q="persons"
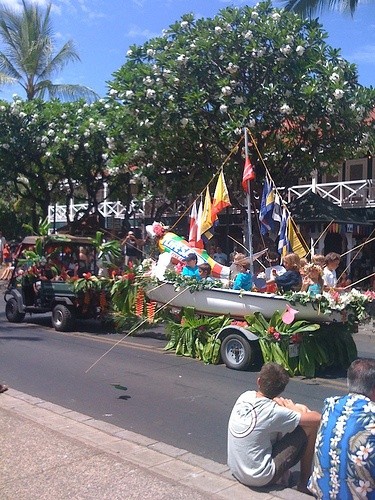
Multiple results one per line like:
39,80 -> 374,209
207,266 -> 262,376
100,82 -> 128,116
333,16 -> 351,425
182,254 -> 214,283
80,247 -> 87,261
230,253 -> 251,291
305,358 -> 375,500
213,247 -> 227,266
227,362 -> 322,496
267,253 -> 302,294
0,231 -> 16,268
123,231 -> 140,265
299,253 -> 351,297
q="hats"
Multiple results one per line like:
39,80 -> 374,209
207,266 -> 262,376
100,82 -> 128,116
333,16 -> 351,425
265,252 -> 279,262
235,260 -> 248,270
182,253 -> 197,261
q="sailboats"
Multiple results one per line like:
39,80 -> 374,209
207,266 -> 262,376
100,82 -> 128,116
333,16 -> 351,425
135,127 -> 355,324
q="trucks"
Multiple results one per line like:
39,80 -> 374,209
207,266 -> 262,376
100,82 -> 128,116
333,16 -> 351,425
4,233 -> 116,333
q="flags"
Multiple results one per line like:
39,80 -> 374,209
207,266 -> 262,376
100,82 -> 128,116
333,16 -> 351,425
259,174 -> 289,266
188,171 -> 232,248
242,157 -> 255,193
